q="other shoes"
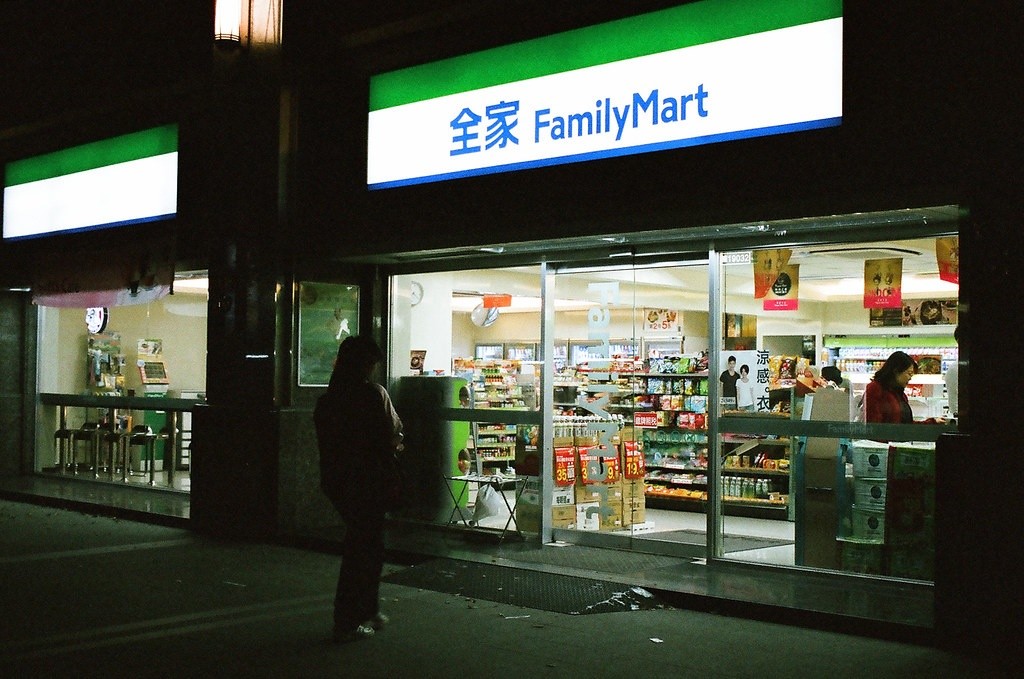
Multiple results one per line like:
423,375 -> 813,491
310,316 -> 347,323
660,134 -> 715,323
362,612 -> 388,628
332,625 -> 375,642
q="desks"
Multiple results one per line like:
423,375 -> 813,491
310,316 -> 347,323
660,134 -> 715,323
443,474 -> 529,547
39,393 -> 203,485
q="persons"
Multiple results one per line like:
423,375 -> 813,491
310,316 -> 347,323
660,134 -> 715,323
735,364 -> 754,411
314,335 -> 404,640
866,351 -> 946,423
720,356 -> 740,409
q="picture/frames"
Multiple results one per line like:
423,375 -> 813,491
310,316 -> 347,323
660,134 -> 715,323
141,361 -> 169,383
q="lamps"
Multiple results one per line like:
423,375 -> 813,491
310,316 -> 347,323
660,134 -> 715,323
334,309 -> 346,326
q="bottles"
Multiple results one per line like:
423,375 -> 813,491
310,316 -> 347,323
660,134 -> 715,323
482,368 -> 504,382
720,476 -> 773,500
553,413 -> 626,438
835,358 -> 886,372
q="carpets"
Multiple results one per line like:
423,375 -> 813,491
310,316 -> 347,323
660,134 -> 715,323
633,528 -> 795,555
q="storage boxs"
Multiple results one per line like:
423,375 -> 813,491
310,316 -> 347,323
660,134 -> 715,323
515,427 -> 645,533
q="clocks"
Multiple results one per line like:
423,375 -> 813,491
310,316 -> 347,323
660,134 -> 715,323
411,281 -> 423,306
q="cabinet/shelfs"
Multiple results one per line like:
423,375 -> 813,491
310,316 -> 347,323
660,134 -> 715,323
454,358 -> 643,488
552,374 -> 793,520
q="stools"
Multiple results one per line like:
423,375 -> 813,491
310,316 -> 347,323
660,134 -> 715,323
73,422 -> 120,478
129,426 -> 178,486
105,424 -> 152,482
55,421 -> 99,476
